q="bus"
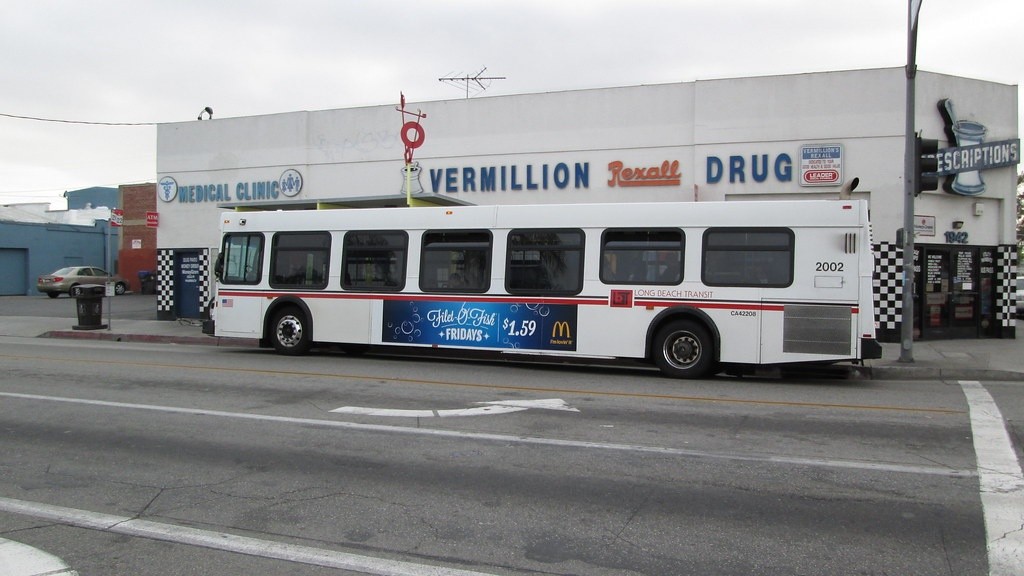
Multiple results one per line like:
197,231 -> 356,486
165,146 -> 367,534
214,199 -> 884,379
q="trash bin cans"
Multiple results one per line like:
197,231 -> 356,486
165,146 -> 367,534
139,271 -> 157,294
71,284 -> 108,329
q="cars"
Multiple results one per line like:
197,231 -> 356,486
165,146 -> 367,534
37,266 -> 130,298
